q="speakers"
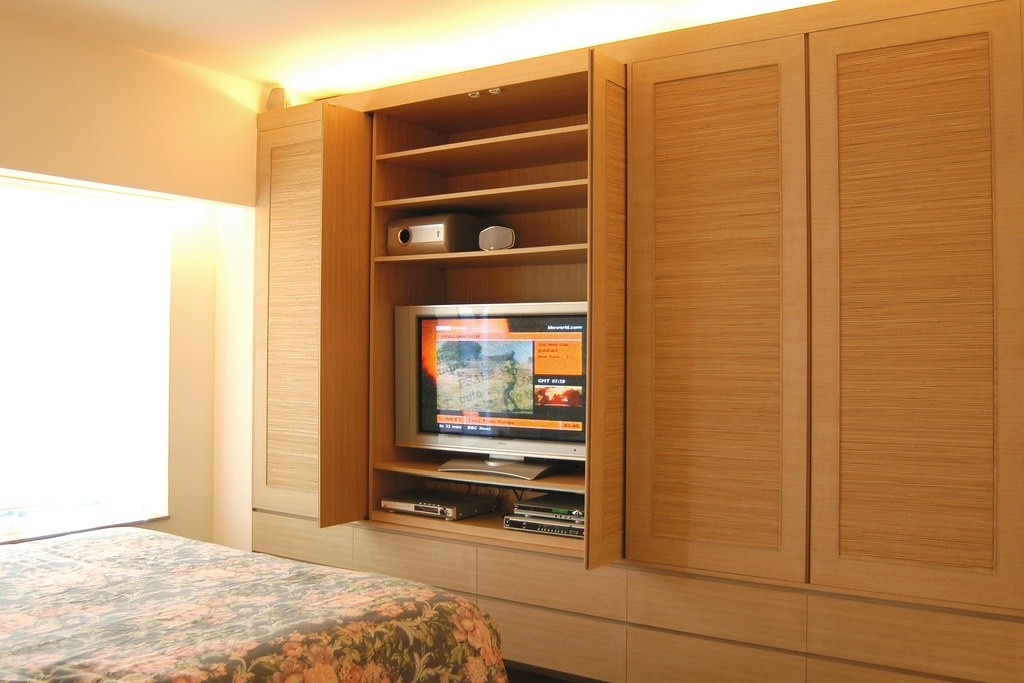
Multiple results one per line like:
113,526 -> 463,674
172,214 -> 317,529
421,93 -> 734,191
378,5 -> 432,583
478,226 -> 515,251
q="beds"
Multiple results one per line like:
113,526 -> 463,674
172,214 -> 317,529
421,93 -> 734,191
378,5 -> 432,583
0,527 -> 506,683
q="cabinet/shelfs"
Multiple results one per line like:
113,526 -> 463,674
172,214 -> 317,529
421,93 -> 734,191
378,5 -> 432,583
251,1 -> 1024,683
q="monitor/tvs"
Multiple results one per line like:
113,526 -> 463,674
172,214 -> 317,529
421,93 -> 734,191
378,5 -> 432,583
393,302 -> 588,480
